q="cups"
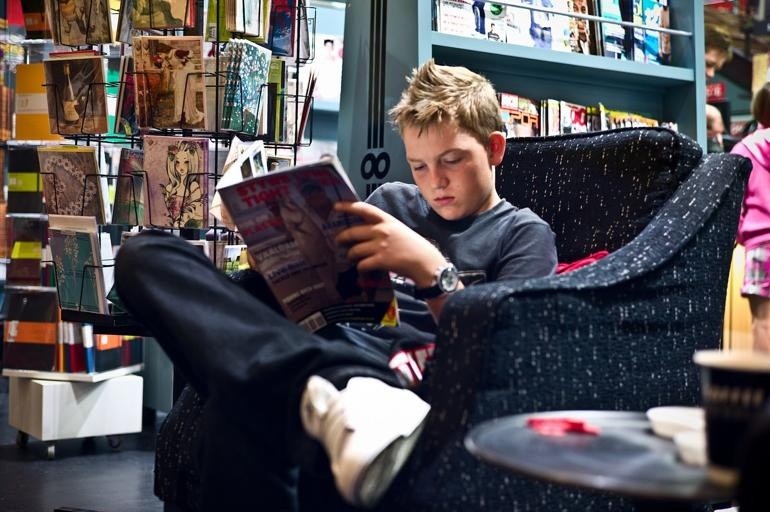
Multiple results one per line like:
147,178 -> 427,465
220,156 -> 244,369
691,348 -> 770,488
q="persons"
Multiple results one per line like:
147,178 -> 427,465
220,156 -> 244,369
702,22 -> 735,148
729,80 -> 770,355
295,174 -> 381,305
114,58 -> 556,510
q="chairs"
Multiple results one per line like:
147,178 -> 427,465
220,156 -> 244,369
149,124 -> 755,509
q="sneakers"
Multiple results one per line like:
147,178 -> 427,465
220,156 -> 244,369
299,372 -> 431,507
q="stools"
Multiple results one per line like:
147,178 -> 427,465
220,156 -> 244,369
7,372 -> 143,461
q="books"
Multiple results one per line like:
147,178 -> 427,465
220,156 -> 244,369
218,140 -> 400,334
433,1 -> 690,146
0,1 -> 318,375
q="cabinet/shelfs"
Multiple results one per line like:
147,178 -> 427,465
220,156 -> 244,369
337,0 -> 710,201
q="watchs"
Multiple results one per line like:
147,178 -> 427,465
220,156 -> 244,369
412,262 -> 459,302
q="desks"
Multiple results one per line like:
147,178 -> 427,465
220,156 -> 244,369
462,407 -> 768,510
